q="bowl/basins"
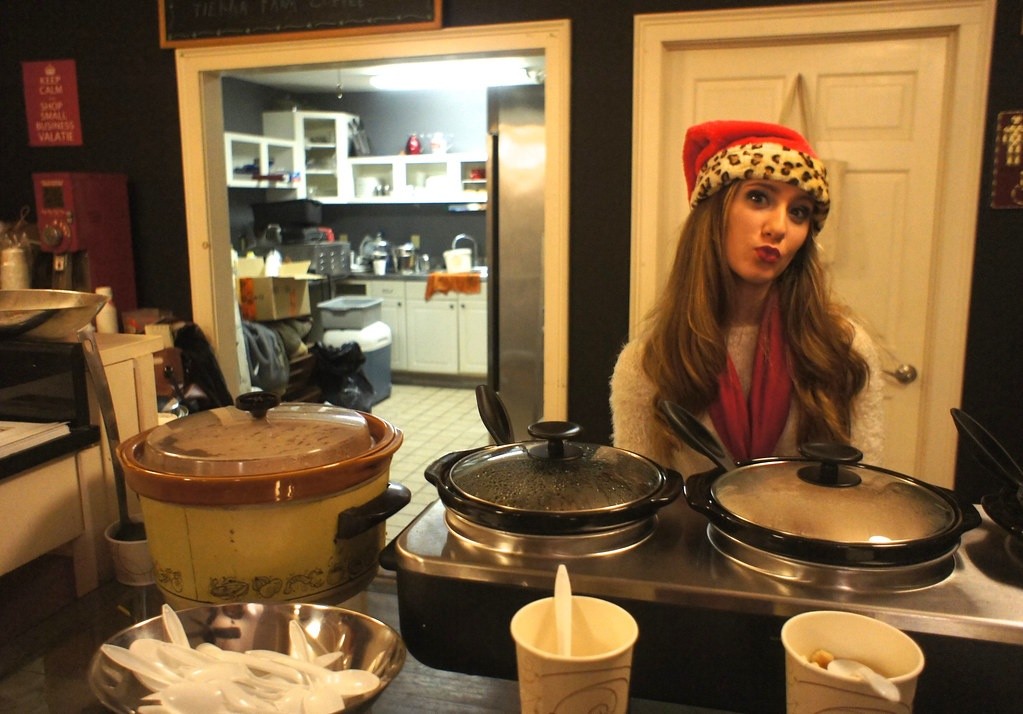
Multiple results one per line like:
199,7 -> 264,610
88,601 -> 407,713
0,289 -> 109,341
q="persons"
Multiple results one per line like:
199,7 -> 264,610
608,122 -> 883,483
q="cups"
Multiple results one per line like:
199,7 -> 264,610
355,177 -> 381,198
781,609 -> 926,714
442,248 -> 472,274
471,169 -> 485,178
510,596 -> 639,714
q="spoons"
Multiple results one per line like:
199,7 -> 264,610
99,603 -> 380,713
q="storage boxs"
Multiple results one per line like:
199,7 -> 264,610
234,255 -> 324,320
122,307 -> 184,395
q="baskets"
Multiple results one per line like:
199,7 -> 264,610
298,241 -> 351,275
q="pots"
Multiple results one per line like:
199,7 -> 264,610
424,383 -> 685,537
657,401 -> 983,568
116,391 -> 413,607
950,407 -> 1023,540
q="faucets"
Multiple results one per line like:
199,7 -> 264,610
450,232 -> 479,267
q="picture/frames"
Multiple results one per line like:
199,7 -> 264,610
158,0 -> 443,51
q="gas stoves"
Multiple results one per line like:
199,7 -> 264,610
377,495 -> 1023,708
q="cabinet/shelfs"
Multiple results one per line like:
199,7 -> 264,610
329,278 -> 405,373
406,280 -> 489,378
224,111 -> 486,207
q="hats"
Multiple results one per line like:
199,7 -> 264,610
682,119 -> 831,237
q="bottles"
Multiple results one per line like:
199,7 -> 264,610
370,231 -> 430,277
407,134 -> 421,154
94,287 -> 120,334
